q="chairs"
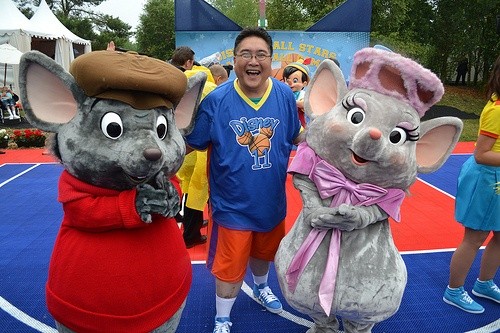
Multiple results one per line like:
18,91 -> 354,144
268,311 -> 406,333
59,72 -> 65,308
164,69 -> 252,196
0,107 -> 22,123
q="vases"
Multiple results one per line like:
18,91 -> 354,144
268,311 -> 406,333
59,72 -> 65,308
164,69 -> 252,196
36,139 -> 44,147
16,140 -> 23,146
24,141 -> 31,147
31,138 -> 36,145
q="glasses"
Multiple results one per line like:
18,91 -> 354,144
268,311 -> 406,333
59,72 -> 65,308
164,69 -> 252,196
237,52 -> 271,60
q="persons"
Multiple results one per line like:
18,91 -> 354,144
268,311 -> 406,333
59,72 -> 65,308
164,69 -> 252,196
281,58 -> 312,130
170,27 -> 303,333
444,62 -> 500,314
0,86 -> 20,120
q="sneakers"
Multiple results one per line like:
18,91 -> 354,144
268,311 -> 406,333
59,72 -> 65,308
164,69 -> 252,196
472,278 -> 500,303
443,284 -> 484,314
213,315 -> 232,333
253,282 -> 282,314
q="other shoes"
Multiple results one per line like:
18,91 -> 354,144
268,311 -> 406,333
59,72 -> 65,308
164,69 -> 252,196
9,114 -> 21,120
186,235 -> 208,249
202,219 -> 209,227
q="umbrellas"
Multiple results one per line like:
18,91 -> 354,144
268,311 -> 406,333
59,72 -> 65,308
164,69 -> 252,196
0,42 -> 26,87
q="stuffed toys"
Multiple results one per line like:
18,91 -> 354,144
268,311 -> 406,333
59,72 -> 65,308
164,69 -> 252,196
19,51 -> 206,332
276,49 -> 464,332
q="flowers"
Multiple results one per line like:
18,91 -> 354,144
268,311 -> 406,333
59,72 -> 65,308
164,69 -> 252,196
12,129 -> 43,138
